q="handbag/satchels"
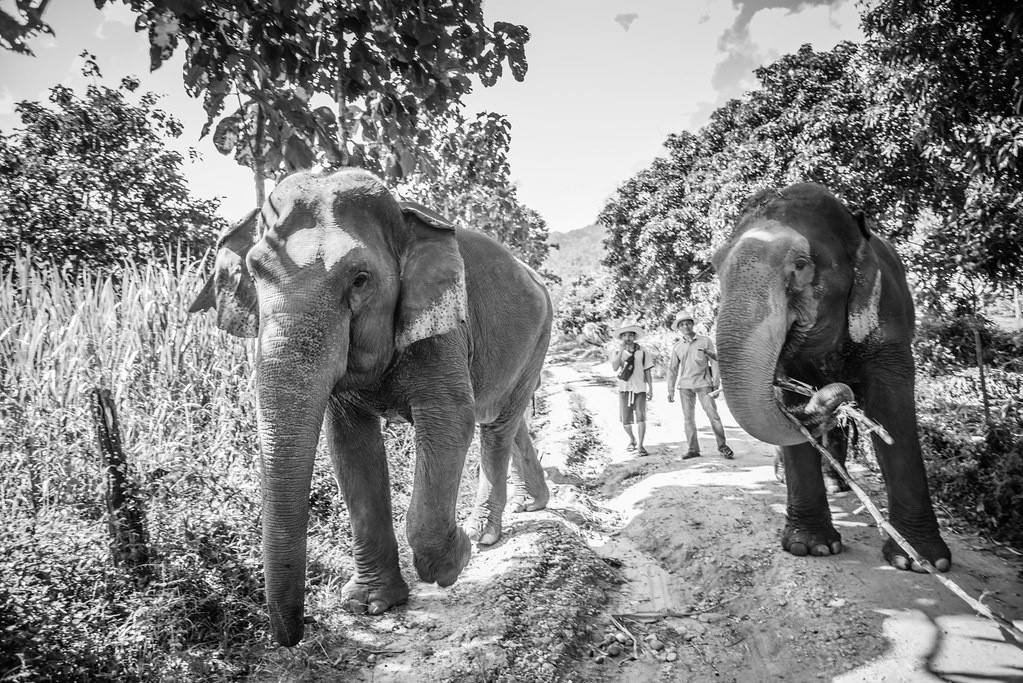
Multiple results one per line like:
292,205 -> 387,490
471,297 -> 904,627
616,356 -> 636,381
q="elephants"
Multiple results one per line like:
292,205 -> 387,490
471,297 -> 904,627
187,171 -> 554,649
711,183 -> 954,573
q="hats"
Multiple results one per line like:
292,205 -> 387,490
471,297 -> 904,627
613,320 -> 645,341
671,310 -> 699,330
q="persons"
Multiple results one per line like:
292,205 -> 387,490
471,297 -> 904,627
667,311 -> 734,459
612,318 -> 655,457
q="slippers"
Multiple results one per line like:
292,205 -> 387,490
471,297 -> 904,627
681,450 -> 700,459
637,446 -> 648,457
719,445 -> 734,458
626,441 -> 637,452
